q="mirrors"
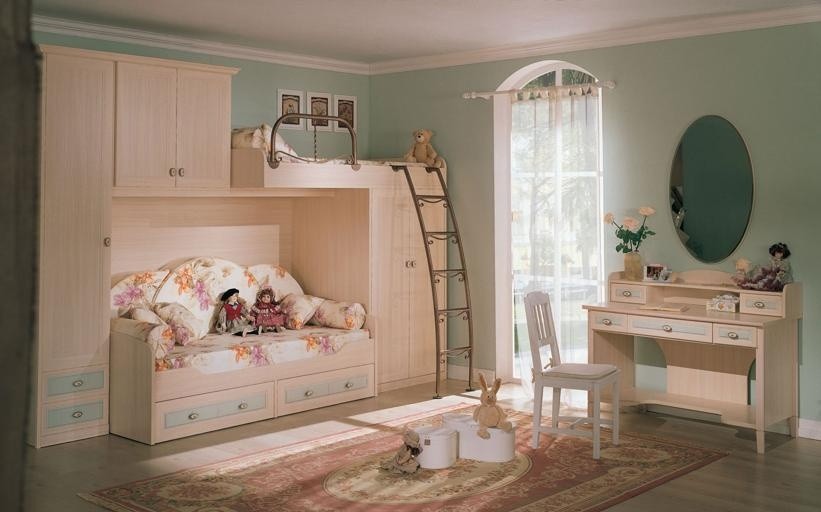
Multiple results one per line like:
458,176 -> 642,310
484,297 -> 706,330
669,114 -> 754,266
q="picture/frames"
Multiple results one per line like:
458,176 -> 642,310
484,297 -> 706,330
276,87 -> 304,130
333,94 -> 358,133
306,91 -> 331,133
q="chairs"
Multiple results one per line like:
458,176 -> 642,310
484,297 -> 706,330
523,290 -> 621,459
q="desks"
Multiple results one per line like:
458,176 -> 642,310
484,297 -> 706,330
582,269 -> 803,455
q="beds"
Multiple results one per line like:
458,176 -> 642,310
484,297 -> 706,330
231,114 -> 445,169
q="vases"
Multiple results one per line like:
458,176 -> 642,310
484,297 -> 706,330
624,253 -> 643,281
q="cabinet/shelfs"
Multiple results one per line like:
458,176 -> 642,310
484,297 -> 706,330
114,50 -> 241,190
24,44 -> 114,450
373,184 -> 445,385
109,341 -> 373,446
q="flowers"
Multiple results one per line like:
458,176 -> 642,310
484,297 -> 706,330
603,205 -> 657,254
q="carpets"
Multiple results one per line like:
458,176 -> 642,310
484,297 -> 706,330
76,400 -> 728,512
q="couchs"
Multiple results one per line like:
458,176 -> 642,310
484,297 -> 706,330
110,257 -> 370,376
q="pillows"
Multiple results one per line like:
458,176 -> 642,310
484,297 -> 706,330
128,304 -> 179,359
277,292 -> 324,332
308,298 -> 365,330
231,123 -> 298,157
154,303 -> 206,344
